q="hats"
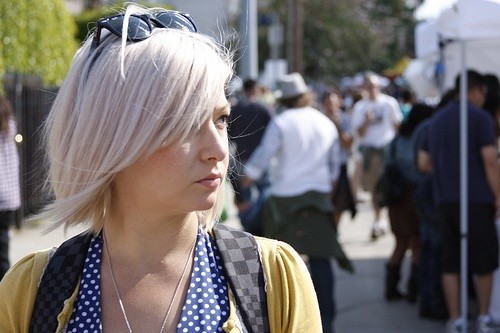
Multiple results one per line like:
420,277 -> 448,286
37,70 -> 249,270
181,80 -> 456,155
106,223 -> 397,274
357,72 -> 381,87
277,70 -> 312,101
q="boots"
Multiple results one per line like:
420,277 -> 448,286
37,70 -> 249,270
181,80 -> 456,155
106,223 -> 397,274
386,264 -> 405,300
405,270 -> 421,304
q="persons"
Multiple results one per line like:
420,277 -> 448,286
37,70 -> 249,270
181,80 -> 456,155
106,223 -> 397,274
320,92 -> 357,228
344,71 -> 404,238
0,89 -> 23,281
227,76 -> 273,229
243,73 -> 342,333
0,4 -> 323,333
373,69 -> 500,333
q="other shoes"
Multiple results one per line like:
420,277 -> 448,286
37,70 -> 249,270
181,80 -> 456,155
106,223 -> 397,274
474,314 -> 498,332
444,317 -> 463,333
371,228 -> 385,239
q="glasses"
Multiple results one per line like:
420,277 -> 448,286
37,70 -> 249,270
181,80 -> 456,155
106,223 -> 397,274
90,11 -> 199,50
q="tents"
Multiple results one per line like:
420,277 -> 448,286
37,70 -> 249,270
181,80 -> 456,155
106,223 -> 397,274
403,0 -> 500,333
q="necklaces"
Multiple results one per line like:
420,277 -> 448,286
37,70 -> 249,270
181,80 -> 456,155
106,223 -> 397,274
102,230 -> 196,333
